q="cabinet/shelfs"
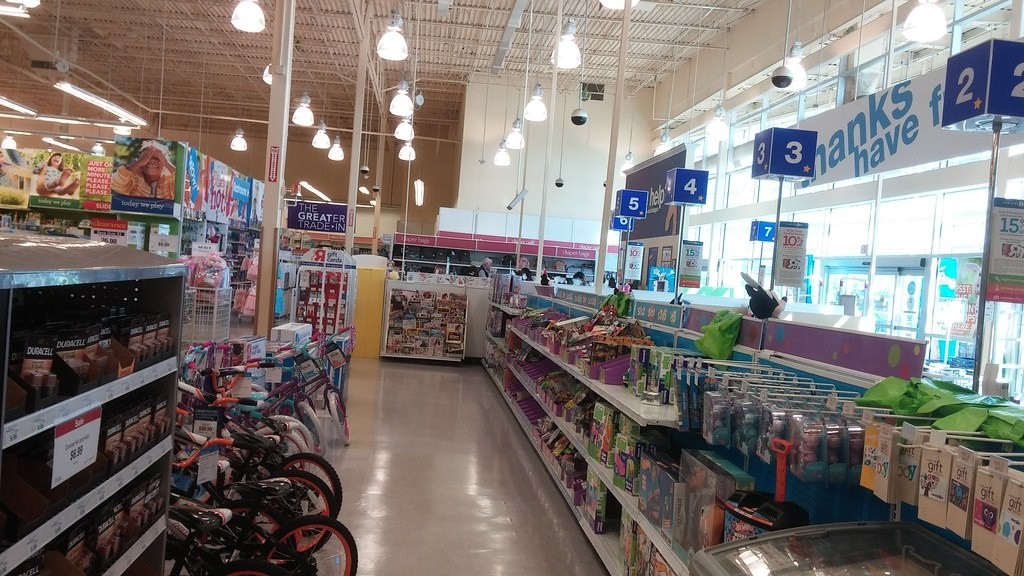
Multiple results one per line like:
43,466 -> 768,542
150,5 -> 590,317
481,273 -> 1024,576
0,136 -> 357,410
0,232 -> 188,576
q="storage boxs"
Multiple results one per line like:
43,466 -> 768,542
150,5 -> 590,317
486,273 -> 528,392
649,543 -> 678,576
613,412 -> 686,550
584,465 -> 607,534
588,401 -> 621,468
627,345 -> 714,406
230,335 -> 266,382
270,322 -> 312,346
616,505 -> 652,576
0,336 -> 173,576
672,449 -> 756,569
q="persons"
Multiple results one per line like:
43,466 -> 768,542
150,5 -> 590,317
473,257 -> 493,278
515,257 -> 533,281
35,153 -> 81,197
110,140 -> 177,200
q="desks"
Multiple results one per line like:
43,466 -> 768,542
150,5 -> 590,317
380,288 -> 469,368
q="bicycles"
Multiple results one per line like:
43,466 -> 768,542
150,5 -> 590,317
162,322 -> 360,576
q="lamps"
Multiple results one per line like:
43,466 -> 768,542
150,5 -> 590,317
494,50 -> 511,166
394,115 -> 414,140
230,47 -> 247,151
902,0 -> 947,42
376,0 -> 409,60
389,60 -> 413,116
705,11 -> 730,141
523,0 -> 547,122
0,0 -> 149,156
328,105 -> 344,161
551,0 -> 581,68
413,144 -> 424,207
620,74 -> 637,179
507,89 -> 524,149
653,6 -> 683,157
782,0 -> 808,92
231,0 -> 265,33
312,60 -> 330,149
291,16 -> 315,126
398,140 -> 416,161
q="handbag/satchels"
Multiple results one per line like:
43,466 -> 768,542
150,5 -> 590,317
599,292 -> 632,316
695,308 -> 745,371
